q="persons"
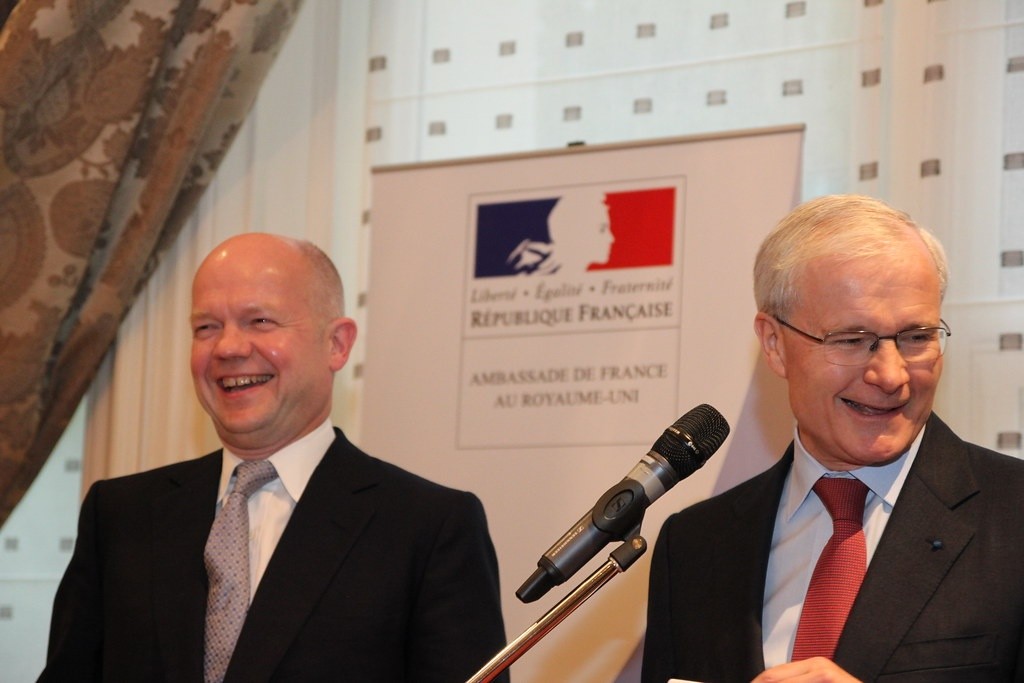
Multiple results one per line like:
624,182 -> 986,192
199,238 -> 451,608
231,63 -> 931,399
34,231 -> 511,682
640,193 -> 1024,682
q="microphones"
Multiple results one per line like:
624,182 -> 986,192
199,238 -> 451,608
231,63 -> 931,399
515,402 -> 730,603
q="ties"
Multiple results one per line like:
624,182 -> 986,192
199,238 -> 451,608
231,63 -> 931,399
792,477 -> 870,661
202,457 -> 279,683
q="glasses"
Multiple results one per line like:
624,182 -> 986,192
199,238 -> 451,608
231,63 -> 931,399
773,311 -> 951,366
